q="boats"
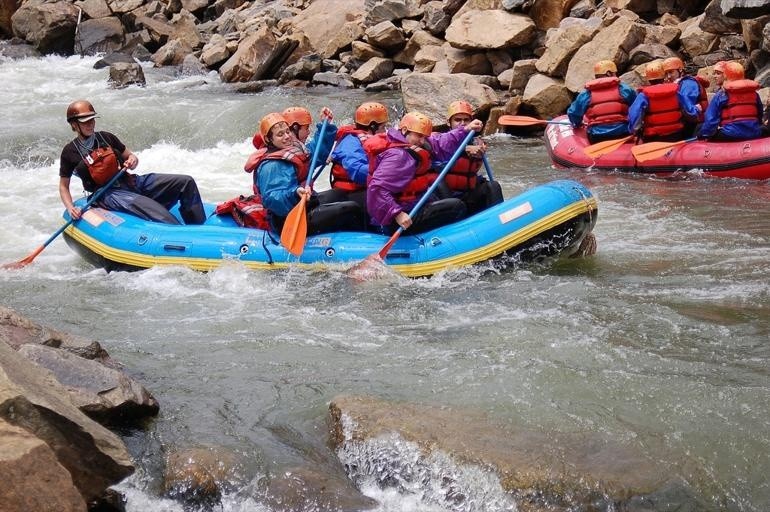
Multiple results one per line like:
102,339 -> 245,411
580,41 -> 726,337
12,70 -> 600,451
543,115 -> 770,182
60,177 -> 600,279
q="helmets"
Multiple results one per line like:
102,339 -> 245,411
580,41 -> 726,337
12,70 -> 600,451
713,60 -> 744,80
282,107 -> 311,127
663,58 -> 684,71
645,61 -> 665,80
67,100 -> 97,121
356,101 -> 388,126
260,113 -> 288,145
398,112 -> 432,137
448,101 -> 473,120
595,60 -> 618,75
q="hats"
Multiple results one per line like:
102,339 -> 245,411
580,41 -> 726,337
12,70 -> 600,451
75,115 -> 100,122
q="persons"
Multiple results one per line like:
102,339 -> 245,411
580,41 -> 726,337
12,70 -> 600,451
565,59 -> 638,142
245,102 -> 503,237
711,59 -> 726,91
699,59 -> 766,142
662,56 -> 711,123
60,100 -> 205,225
628,59 -> 702,139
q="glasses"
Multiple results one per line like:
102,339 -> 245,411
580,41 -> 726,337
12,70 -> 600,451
453,117 -> 471,122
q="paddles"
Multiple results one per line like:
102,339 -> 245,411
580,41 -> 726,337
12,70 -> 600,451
278,107 -> 334,258
632,134 -> 698,163
497,114 -> 587,126
1,155 -> 129,273
585,131 -> 639,160
346,128 -> 476,288
